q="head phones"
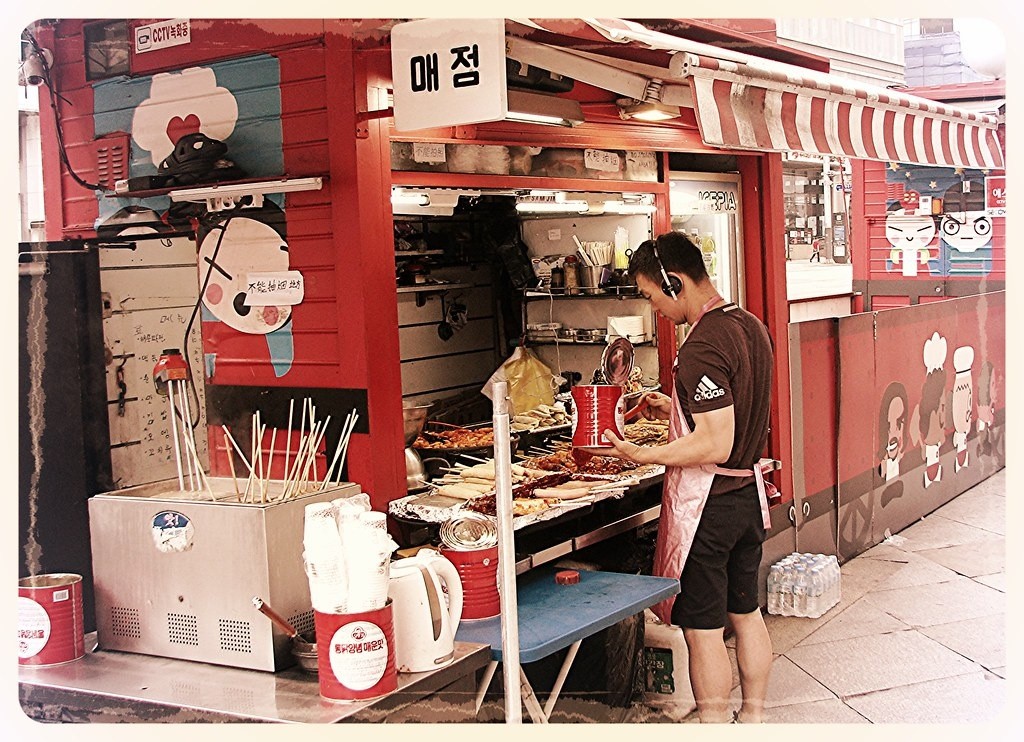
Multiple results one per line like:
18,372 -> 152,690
652,242 -> 683,301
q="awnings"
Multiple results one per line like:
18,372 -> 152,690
507,18 -> 1005,170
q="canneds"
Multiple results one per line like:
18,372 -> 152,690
18,573 -> 87,667
570,338 -> 634,448
437,511 -> 502,623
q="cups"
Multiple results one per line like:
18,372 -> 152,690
18,573 -> 85,666
314,597 -> 399,702
305,498 -> 389,612
579,262 -> 612,293
614,268 -> 638,293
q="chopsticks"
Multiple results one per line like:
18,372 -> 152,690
572,234 -> 614,266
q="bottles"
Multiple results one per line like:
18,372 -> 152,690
678,228 -> 717,277
549,253 -> 578,297
417,238 -> 428,253
767,551 -> 843,618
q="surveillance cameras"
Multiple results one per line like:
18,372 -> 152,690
25,60 -> 46,86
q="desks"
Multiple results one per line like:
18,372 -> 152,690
18,642 -> 493,724
455,566 -> 681,723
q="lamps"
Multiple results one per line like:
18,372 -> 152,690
626,103 -> 681,121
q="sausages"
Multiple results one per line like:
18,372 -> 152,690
532,480 -> 610,499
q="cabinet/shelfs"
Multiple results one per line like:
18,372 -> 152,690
519,289 -> 654,348
395,248 -> 473,294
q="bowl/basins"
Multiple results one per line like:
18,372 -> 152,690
401,400 -> 435,448
291,631 -> 318,674
528,324 -> 607,342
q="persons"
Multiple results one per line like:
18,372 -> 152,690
809,238 -> 821,263
580,230 -> 782,722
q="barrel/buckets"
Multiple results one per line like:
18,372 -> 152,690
643,617 -> 697,712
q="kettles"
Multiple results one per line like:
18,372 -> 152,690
387,555 -> 463,670
